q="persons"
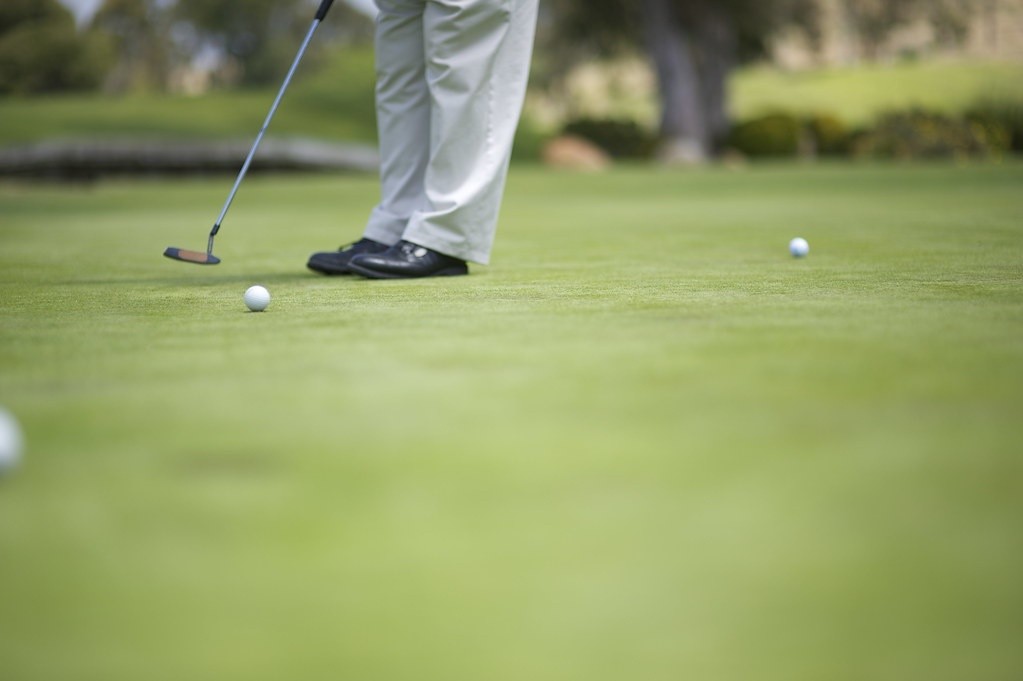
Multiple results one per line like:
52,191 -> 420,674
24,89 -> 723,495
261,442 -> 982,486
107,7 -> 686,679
637,0 -> 728,166
306,0 -> 541,279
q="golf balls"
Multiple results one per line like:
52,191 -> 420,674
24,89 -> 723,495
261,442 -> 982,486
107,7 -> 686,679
789,237 -> 810,257
244,285 -> 270,312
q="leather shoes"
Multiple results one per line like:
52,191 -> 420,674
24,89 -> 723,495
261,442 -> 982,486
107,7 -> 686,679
346,239 -> 467,279
307,237 -> 391,275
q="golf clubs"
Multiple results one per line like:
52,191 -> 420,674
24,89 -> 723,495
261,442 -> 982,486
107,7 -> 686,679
163,0 -> 333,266
